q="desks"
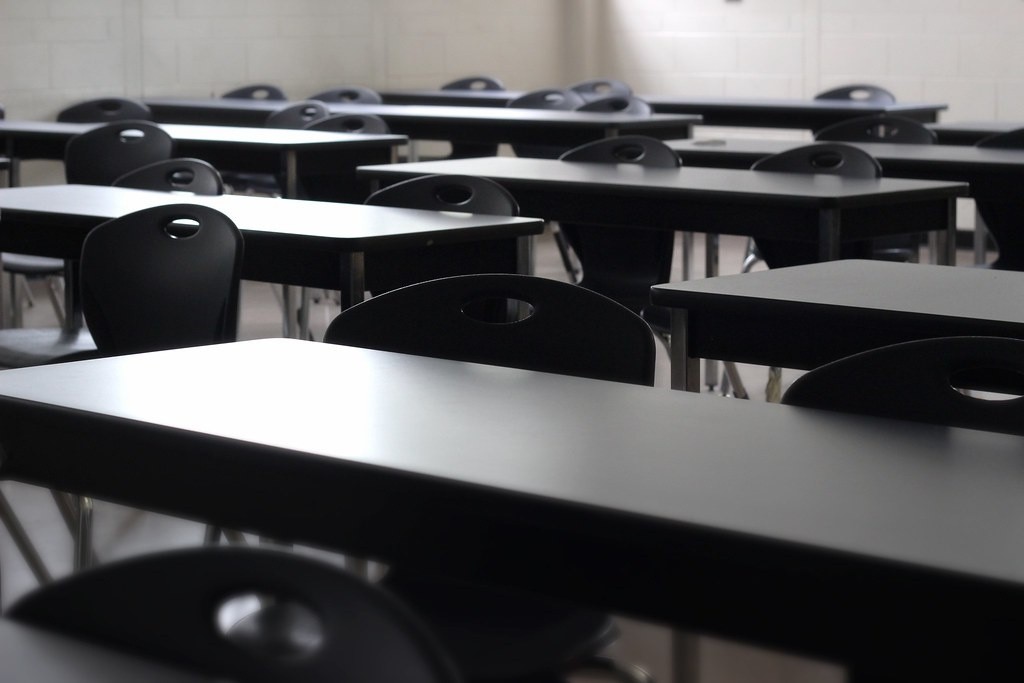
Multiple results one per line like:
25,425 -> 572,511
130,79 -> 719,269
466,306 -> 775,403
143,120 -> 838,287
0,86 -> 1024,683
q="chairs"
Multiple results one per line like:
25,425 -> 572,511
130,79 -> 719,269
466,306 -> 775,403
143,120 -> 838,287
308,85 -> 384,104
52,204 -> 248,572
572,79 -> 633,95
967,128 -> 1024,272
779,332 -> 1024,436
507,87 -> 603,160
529,134 -> 682,362
363,174 -> 522,217
721,142 -> 883,404
813,114 -> 937,262
320,273 -> 655,683
270,100 -> 329,127
0,119 -> 175,330
576,92 -> 651,118
59,98 -> 153,121
3,545 -> 465,683
302,113 -> 391,134
398,76 -> 507,162
0,158 -> 225,364
815,85 -> 898,106
222,84 -> 289,199
